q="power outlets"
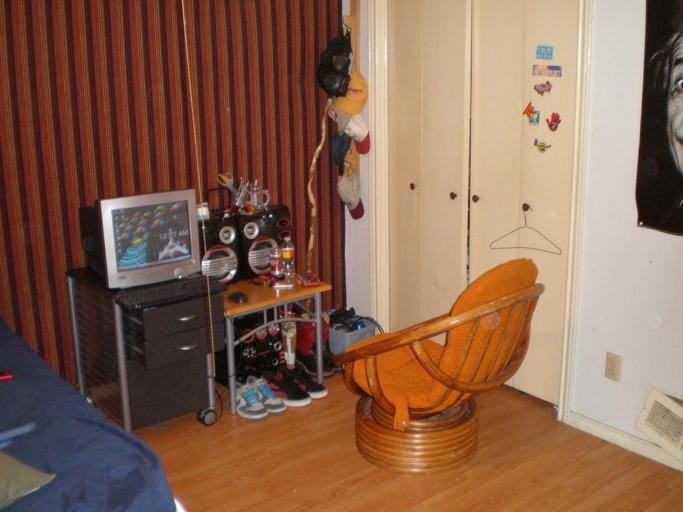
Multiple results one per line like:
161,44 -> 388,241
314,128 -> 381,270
604,352 -> 621,381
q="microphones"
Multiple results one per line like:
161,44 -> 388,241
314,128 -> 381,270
78,187 -> 201,290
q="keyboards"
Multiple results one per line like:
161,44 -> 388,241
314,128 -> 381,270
114,275 -> 215,310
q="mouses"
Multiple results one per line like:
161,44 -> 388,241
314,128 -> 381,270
229,291 -> 248,304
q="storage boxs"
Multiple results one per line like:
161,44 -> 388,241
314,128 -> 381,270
329,317 -> 377,355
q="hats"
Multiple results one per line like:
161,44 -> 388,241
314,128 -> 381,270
317,34 -> 370,220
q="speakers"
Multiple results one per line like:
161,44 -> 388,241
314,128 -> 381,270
238,204 -> 291,280
199,207 -> 243,285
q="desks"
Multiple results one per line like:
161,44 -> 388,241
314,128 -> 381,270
216,271 -> 334,414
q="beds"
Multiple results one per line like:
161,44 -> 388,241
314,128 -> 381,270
1,317 -> 180,512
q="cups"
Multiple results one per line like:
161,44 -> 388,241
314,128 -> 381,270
232,191 -> 271,213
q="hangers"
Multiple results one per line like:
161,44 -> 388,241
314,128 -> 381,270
488,203 -> 563,257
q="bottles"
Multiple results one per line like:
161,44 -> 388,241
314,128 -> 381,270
270,237 -> 296,282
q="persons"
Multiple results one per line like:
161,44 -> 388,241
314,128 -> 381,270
646,29 -> 683,173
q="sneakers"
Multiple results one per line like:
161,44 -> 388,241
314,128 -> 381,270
297,349 -> 341,378
263,369 -> 311,409
248,377 -> 286,414
280,364 -> 328,400
234,382 -> 268,420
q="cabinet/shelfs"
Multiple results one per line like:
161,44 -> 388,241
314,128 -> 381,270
63,265 -> 226,439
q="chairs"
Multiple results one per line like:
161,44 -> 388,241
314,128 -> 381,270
329,257 -> 546,476
